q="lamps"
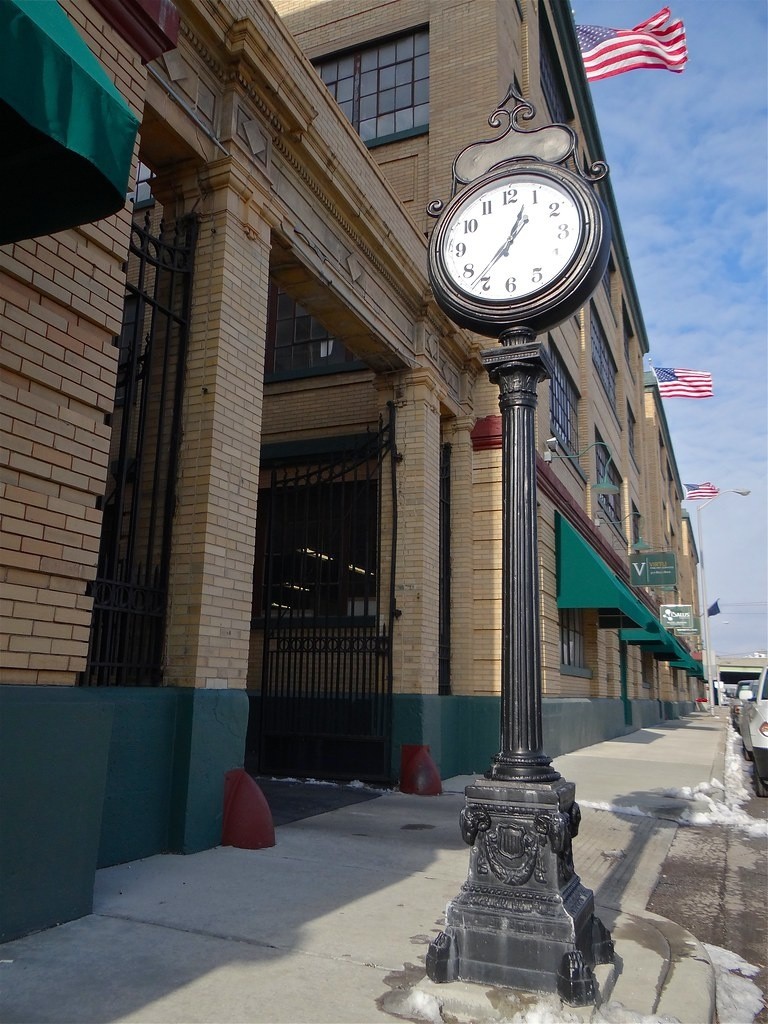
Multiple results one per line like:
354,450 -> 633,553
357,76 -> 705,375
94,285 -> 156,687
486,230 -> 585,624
594,512 -> 651,550
544,442 -> 619,496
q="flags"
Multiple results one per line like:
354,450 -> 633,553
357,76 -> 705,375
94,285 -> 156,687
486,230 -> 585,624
683,482 -> 718,500
652,367 -> 714,398
576,6 -> 689,83
707,602 -> 720,616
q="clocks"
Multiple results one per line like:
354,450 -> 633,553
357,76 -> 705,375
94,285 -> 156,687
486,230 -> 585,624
427,67 -> 612,338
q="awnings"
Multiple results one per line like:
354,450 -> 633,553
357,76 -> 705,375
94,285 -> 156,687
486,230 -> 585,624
553,510 -> 703,677
0,0 -> 141,248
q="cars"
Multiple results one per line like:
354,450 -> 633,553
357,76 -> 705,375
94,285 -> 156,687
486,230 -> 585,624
728,664 -> 768,797
